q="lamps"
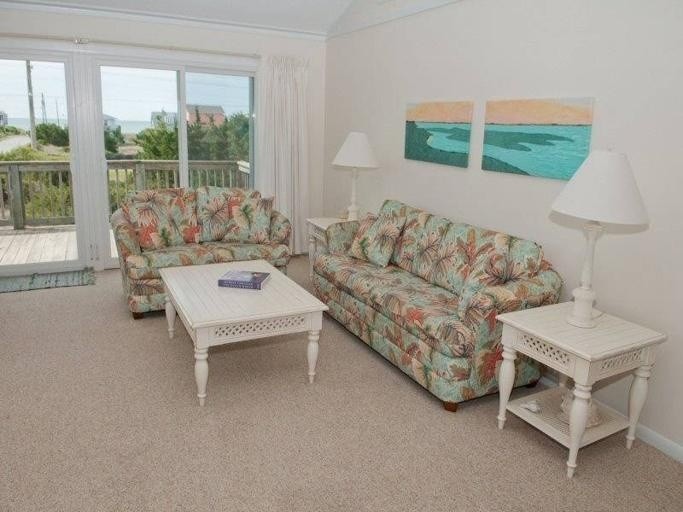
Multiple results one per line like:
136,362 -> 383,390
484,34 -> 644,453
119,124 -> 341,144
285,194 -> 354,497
549,147 -> 656,330
329,130 -> 381,224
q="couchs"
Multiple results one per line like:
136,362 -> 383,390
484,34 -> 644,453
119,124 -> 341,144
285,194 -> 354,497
110,182 -> 292,321
310,196 -> 563,420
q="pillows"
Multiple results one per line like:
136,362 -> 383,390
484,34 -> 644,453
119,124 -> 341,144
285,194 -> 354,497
347,213 -> 405,267
456,249 -> 512,322
125,197 -> 185,252
221,192 -> 276,245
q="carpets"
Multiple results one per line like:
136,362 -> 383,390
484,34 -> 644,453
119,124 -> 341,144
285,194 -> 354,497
1,264 -> 97,292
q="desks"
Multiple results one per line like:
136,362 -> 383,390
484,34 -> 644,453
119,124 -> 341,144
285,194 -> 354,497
303,215 -> 351,281
152,259 -> 331,408
491,300 -> 667,478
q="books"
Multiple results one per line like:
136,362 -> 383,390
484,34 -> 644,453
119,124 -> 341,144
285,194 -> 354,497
218,270 -> 272,290
